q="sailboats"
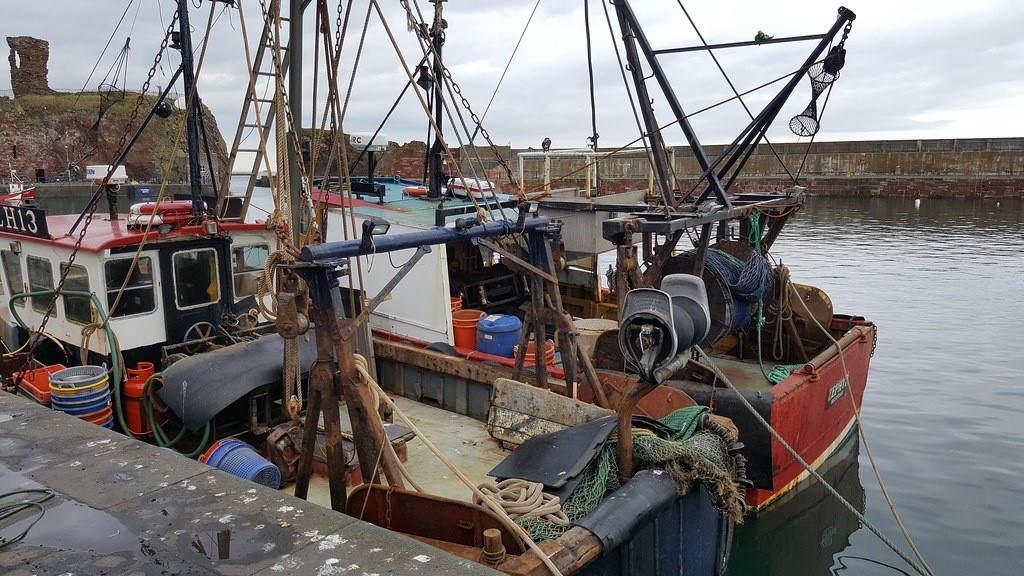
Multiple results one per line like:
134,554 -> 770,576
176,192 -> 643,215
1,2 -> 877,576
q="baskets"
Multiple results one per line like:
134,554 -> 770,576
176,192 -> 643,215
198,438 -> 282,491
48,363 -> 114,431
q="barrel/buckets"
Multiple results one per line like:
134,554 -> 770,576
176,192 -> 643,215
476,314 -> 522,358
513,339 -> 556,367
452,309 -> 487,351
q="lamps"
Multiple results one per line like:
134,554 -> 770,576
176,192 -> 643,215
364,221 -> 390,234
525,201 -> 540,212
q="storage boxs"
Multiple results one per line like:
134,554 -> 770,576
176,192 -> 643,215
13,364 -> 67,408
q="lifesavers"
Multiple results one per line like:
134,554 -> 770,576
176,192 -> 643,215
402,185 -> 448,196
128,199 -> 208,225
